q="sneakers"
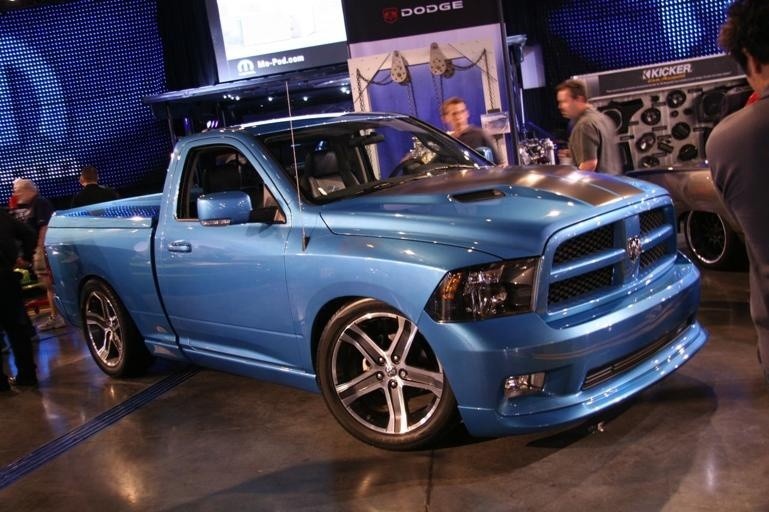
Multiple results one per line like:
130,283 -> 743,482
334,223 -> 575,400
1,376 -> 39,392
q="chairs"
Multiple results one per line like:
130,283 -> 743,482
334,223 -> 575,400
199,151 -> 360,227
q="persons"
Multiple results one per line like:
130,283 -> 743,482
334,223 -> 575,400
442,96 -> 503,166
0,179 -> 66,392
69,165 -> 121,210
705,1 -> 767,381
553,81 -> 620,176
